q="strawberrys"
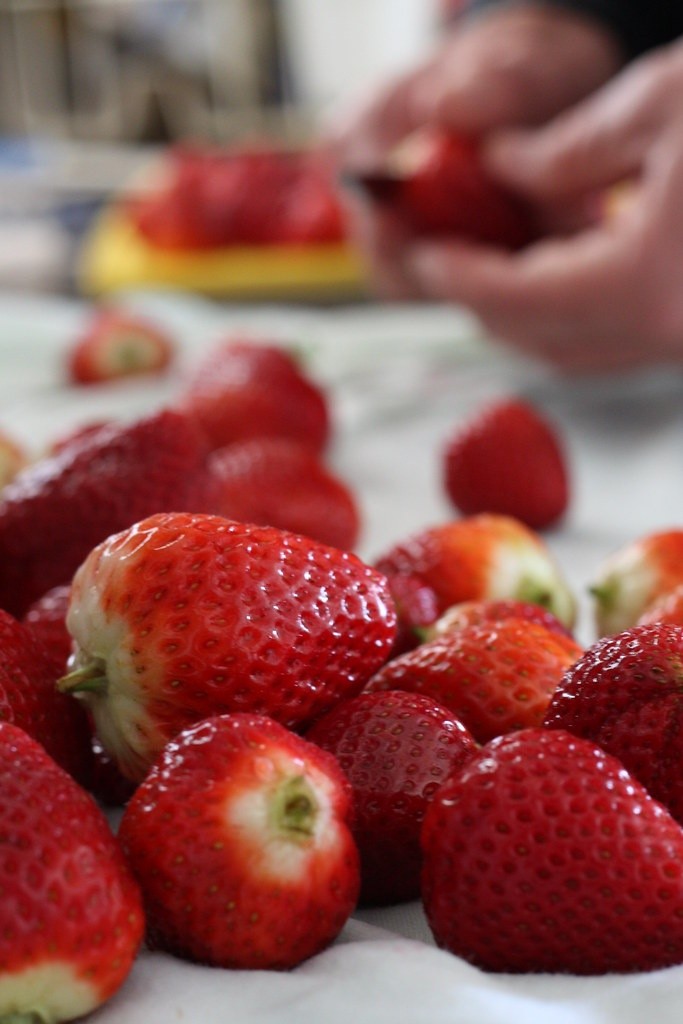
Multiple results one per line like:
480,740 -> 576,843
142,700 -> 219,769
0,130 -> 683,1024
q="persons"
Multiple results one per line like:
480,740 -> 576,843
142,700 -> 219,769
339,0 -> 683,378
